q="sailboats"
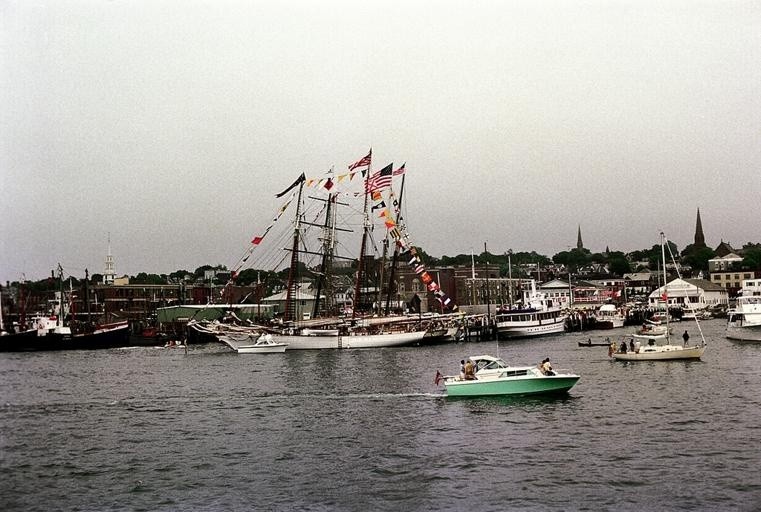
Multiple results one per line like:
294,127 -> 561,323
0,263 -> 168,352
177,146 -> 468,349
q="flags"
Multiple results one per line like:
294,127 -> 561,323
348,152 -> 372,170
325,177 -> 334,191
364,162 -> 392,195
252,237 -> 263,244
393,163 -> 406,177
371,190 -> 460,312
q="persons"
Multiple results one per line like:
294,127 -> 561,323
682,330 -> 690,348
538,359 -> 548,375
460,359 -> 466,378
607,338 -> 642,356
544,357 -> 556,376
464,360 -> 478,380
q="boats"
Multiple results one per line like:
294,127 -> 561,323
495,279 -> 565,338
435,355 -> 580,397
237,333 -> 291,354
578,232 -> 761,361
